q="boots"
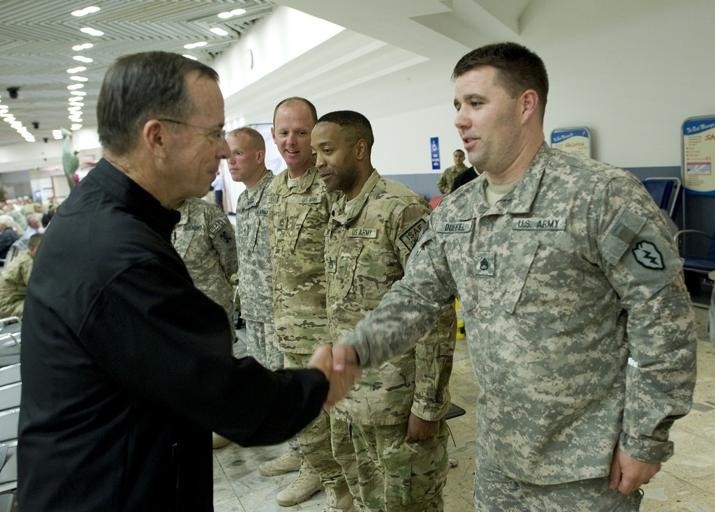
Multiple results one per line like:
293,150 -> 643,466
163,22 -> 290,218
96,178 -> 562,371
258,446 -> 302,477
274,461 -> 324,507
321,478 -> 358,512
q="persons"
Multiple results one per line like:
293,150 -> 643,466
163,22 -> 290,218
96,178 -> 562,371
436,148 -> 468,195
0,231 -> 45,326
11,214 -> 45,258
321,42 -> 699,512
15,50 -> 360,512
258,96 -> 342,508
0,214 -> 19,267
309,110 -> 457,512
225,125 -> 284,372
171,196 -> 240,450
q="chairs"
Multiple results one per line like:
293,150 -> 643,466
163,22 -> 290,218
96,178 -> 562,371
673,231 -> 715,345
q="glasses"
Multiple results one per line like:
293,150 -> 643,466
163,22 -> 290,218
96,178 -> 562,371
156,117 -> 226,143
